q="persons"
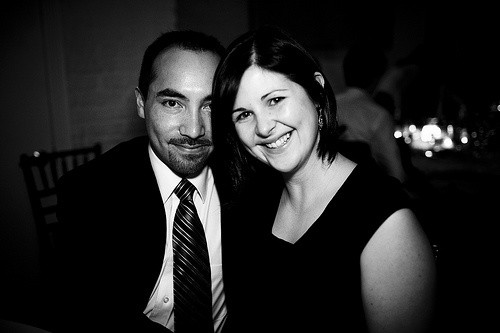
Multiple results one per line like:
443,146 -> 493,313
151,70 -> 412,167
335,52 -> 406,186
51,30 -> 239,333
212,31 -> 436,333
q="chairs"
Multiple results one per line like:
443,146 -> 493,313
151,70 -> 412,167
18,142 -> 114,330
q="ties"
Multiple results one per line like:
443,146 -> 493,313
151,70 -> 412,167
172,178 -> 215,333
